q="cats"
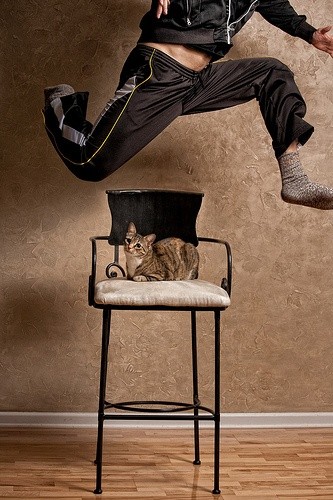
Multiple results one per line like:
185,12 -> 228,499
124,221 -> 201,283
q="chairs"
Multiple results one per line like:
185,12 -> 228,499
87,188 -> 232,495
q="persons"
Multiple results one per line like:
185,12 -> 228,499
41,0 -> 333,212
